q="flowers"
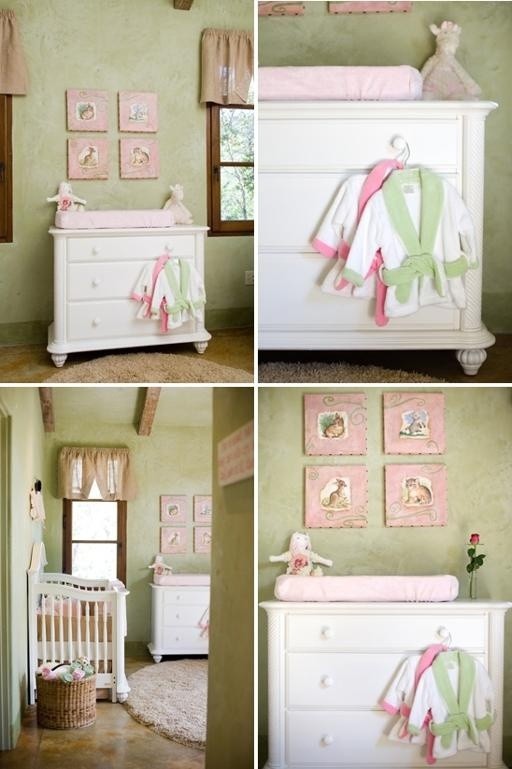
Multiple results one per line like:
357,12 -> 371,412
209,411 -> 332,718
466,533 -> 486,598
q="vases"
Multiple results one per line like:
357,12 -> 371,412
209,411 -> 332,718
469,570 -> 480,598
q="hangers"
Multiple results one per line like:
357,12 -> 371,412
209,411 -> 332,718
161,243 -> 180,265
384,136 -> 418,187
434,628 -> 460,671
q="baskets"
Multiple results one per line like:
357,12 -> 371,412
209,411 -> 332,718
36,670 -> 97,730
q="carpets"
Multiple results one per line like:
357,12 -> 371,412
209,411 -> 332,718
121,659 -> 208,750
259,362 -> 448,382
41,354 -> 253,382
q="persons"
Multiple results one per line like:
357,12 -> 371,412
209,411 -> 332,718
419,20 -> 481,100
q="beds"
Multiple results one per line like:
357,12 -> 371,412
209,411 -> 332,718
27,541 -> 131,705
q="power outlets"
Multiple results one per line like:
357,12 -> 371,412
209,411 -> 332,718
245,271 -> 254,285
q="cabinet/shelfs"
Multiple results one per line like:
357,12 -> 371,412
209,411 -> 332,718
148,582 -> 211,664
259,100 -> 500,376
259,601 -> 511,768
46,225 -> 211,368
